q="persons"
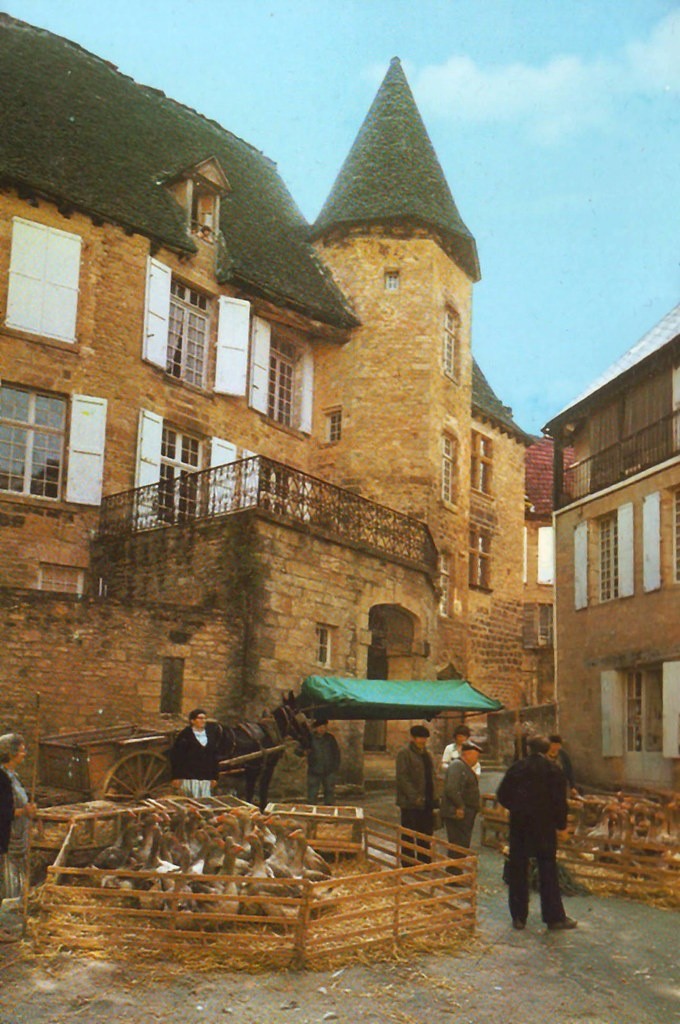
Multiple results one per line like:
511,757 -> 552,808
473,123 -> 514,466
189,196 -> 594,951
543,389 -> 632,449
0,733 -> 39,907
441,725 -> 482,776
171,709 -> 220,799
394,725 -> 440,873
295,717 -> 341,805
497,733 -> 579,929
545,736 -> 579,800
439,741 -> 484,887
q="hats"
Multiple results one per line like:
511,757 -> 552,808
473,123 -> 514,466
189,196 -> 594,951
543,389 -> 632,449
461,740 -> 484,754
410,725 -> 430,738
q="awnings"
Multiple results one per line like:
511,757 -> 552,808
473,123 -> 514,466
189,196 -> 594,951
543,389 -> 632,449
302,675 -> 505,722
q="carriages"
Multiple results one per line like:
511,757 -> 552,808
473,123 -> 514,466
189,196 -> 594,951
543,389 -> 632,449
38,690 -> 314,814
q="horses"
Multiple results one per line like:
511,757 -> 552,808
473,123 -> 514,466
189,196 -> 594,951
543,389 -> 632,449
219,689 -> 314,816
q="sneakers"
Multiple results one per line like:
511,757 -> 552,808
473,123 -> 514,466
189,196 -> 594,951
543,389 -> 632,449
511,917 -> 527,928
547,917 -> 577,930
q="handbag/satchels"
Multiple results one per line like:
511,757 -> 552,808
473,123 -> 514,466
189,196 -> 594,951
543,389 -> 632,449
432,808 -> 444,830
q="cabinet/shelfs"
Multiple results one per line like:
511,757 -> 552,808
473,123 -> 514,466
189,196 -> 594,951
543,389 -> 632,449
263,802 -> 365,863
147,794 -> 261,828
479,792 -> 510,848
31,800 -> 155,853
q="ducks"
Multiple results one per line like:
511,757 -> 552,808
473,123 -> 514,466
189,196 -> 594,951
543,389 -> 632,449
90,807 -> 332,931
559,788 -> 680,872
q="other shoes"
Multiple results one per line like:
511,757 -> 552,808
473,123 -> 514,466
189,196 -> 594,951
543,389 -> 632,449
0,929 -> 17,943
